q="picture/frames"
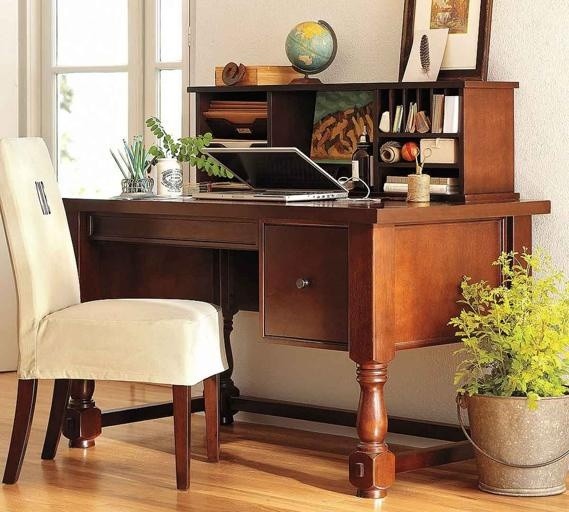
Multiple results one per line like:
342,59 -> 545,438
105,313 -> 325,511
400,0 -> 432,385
398,0 -> 493,83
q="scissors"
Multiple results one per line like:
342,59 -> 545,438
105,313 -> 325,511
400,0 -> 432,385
411,147 -> 432,174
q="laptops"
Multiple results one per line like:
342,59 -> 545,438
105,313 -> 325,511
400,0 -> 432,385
192,147 -> 350,203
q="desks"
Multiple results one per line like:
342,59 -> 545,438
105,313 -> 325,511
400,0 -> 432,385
58,195 -> 552,499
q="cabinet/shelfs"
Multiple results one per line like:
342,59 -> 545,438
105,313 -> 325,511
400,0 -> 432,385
185,80 -> 522,205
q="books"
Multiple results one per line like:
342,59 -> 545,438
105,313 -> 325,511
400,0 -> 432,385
382,175 -> 464,195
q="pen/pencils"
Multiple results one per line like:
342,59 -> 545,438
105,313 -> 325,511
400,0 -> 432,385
109,134 -> 159,194
418,163 -> 423,174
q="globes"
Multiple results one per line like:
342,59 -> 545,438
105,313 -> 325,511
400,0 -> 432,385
284,19 -> 337,85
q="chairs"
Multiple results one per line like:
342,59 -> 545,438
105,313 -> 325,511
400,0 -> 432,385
1,135 -> 228,492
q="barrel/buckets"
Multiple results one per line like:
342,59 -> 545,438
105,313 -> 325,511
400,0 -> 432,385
454,383 -> 569,497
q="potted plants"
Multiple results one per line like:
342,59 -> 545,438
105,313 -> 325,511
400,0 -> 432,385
109,116 -> 236,200
447,245 -> 569,498
